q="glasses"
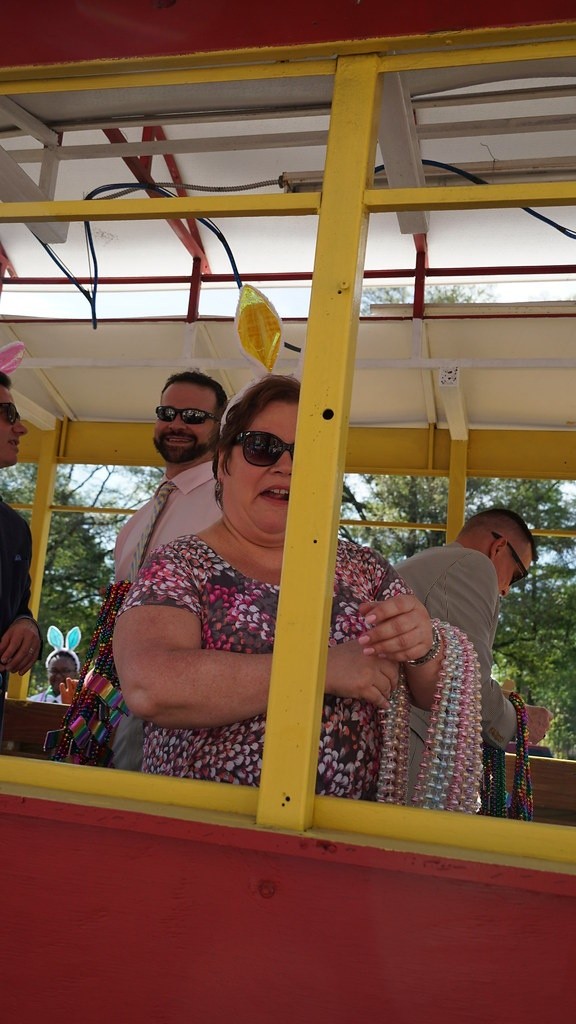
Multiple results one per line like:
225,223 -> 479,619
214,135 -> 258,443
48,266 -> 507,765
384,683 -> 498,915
232,431 -> 294,466
491,531 -> 528,585
1,403 -> 21,424
155,405 -> 219,424
47,668 -> 76,677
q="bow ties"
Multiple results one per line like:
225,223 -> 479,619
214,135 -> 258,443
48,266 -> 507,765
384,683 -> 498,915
46,694 -> 62,703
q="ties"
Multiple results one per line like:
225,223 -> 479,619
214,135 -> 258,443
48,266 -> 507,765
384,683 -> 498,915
126,481 -> 178,584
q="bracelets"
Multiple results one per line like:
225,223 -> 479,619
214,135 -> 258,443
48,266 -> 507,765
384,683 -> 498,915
407,626 -> 441,669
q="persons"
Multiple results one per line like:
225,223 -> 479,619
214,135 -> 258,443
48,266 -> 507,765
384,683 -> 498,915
0,372 -> 42,753
28,652 -> 85,706
111,373 -> 443,799
114,370 -> 229,773
388,506 -> 556,808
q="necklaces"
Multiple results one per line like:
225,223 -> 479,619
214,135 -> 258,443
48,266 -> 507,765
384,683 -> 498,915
375,619 -> 484,814
489,689 -> 534,821
53,580 -> 135,766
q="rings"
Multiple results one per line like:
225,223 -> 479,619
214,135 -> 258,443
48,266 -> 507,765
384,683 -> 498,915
29,647 -> 34,654
